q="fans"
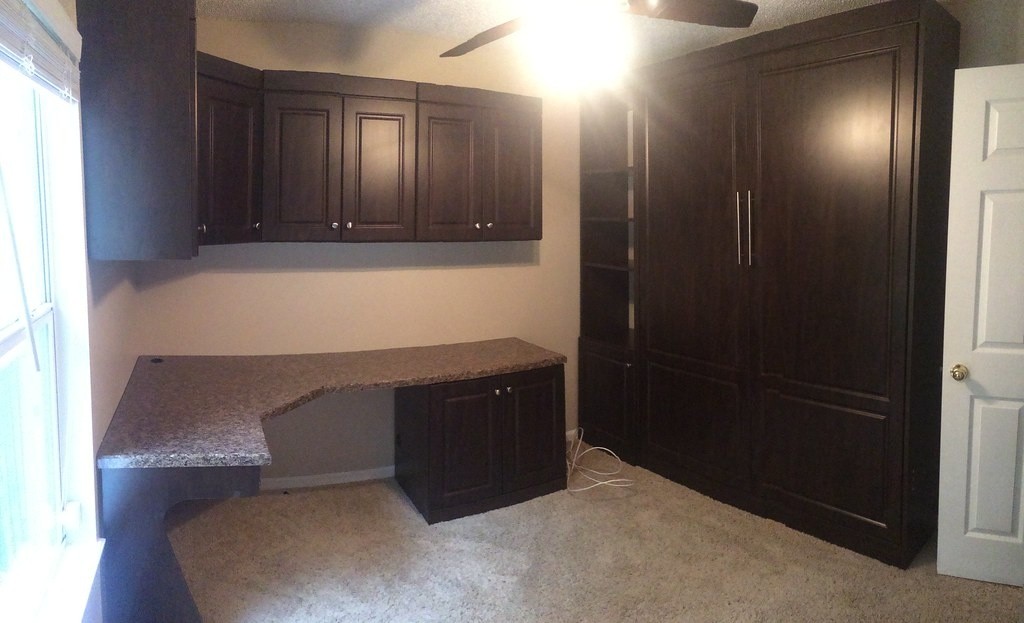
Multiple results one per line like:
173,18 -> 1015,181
437,0 -> 759,58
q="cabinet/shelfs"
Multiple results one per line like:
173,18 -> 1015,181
260,70 -> 419,245
391,363 -> 569,524
631,1 -> 962,571
417,81 -> 543,243
195,50 -> 264,247
578,69 -> 640,467
